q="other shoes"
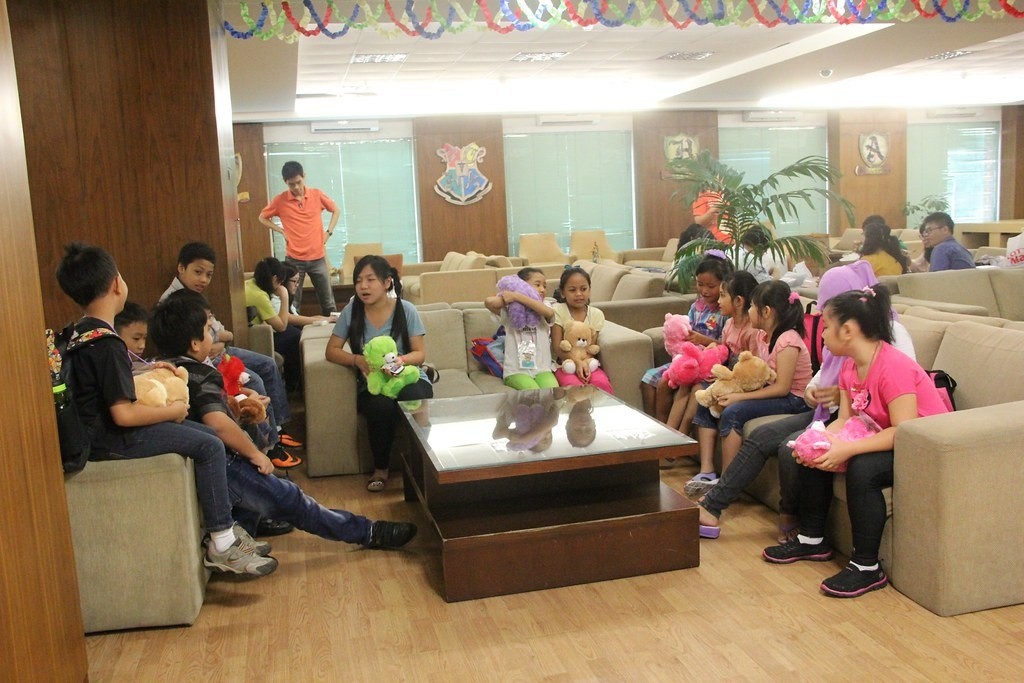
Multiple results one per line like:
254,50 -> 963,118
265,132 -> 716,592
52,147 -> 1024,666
368,470 -> 390,490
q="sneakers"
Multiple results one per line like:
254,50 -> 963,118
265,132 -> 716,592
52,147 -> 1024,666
276,429 -> 303,449
256,515 -> 293,535
762,535 -> 832,564
820,562 -> 888,598
267,447 -> 304,470
202,533 -> 278,578
368,520 -> 416,548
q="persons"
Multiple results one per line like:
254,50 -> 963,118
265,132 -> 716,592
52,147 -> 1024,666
640,175 -> 976,597
492,390 -> 559,453
554,387 -> 597,447
46,239 -> 416,577
550,267 -> 613,394
485,267 -> 560,390
325,253 -> 433,491
406,399 -> 430,442
258,161 -> 340,314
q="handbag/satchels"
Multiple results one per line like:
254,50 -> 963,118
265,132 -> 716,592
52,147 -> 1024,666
470,325 -> 506,379
1007,230 -> 1024,267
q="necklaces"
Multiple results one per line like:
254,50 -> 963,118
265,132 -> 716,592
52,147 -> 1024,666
850,340 -> 880,393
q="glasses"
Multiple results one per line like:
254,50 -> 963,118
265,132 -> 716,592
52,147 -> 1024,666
288,279 -> 300,284
924,227 -> 944,233
919,233 -> 929,240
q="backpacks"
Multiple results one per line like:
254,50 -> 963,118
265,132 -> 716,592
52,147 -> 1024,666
925,368 -> 957,413
43,323 -> 131,472
800,300 -> 827,377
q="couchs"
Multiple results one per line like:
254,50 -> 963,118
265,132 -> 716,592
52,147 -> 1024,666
64,227 -> 1023,635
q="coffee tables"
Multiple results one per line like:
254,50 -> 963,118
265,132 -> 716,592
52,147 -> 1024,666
397,384 -> 702,605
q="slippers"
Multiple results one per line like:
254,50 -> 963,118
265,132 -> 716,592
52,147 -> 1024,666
699,524 -> 720,539
777,522 -> 802,546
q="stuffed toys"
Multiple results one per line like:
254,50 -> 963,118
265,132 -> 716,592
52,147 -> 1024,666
506,404 -> 546,450
362,335 -> 420,398
496,274 -> 542,329
663,342 -> 729,387
132,366 -> 189,407
786,416 -> 875,473
227,396 -> 266,424
216,353 -> 250,395
663,313 -> 704,359
559,319 -> 600,374
400,399 -> 421,411
695,351 -> 777,417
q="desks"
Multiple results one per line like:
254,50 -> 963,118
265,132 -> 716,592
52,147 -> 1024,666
300,278 -> 354,319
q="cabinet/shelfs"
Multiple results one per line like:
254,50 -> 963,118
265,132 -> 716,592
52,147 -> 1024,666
952,222 -> 1024,249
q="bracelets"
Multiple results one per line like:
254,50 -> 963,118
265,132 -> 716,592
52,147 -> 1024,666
353,354 -> 359,368
327,229 -> 332,235
400,355 -> 406,365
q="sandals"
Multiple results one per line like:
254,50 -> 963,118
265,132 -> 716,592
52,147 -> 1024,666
681,471 -> 720,495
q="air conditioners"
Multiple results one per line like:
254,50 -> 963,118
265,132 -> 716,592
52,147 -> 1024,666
539,114 -> 602,128
744,111 -> 796,122
927,107 -> 982,120
310,121 -> 379,133
